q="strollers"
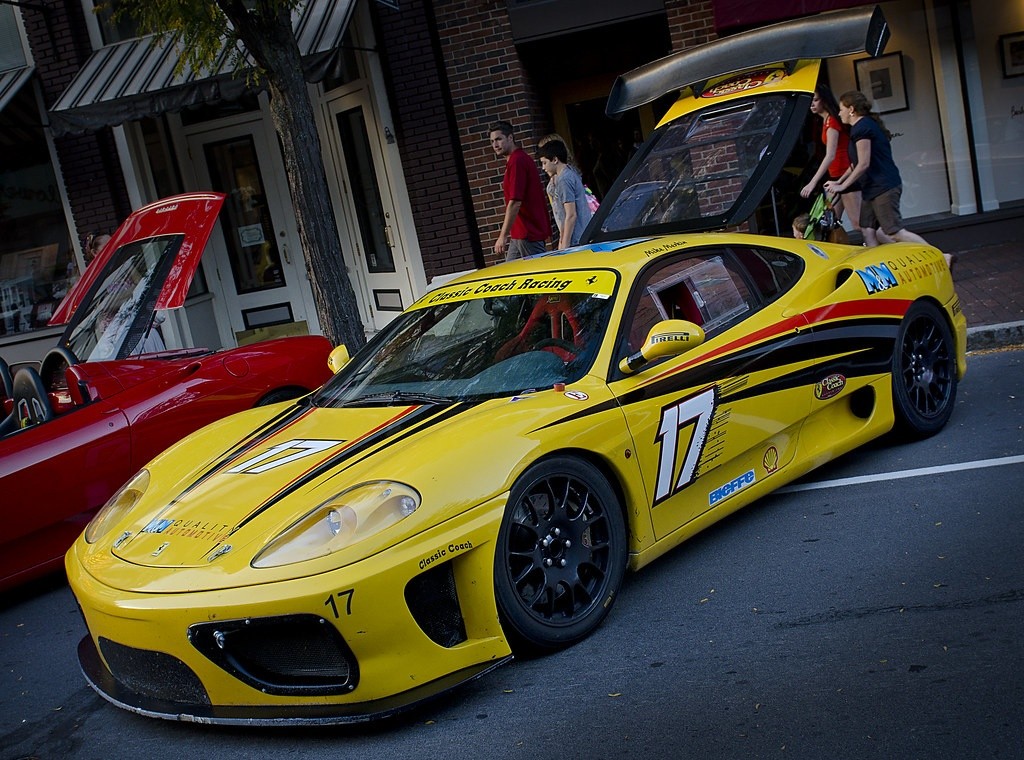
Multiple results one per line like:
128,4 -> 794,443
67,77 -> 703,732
796,186 -> 848,244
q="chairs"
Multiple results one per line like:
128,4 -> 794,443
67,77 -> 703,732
0,356 -> 51,438
494,296 -> 583,369
627,276 -> 713,352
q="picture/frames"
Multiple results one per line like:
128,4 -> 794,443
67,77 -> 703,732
853,50 -> 909,116
999,31 -> 1024,79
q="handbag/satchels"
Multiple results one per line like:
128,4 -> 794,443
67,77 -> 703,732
565,162 -> 600,216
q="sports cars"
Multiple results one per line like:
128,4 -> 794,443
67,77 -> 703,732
64,5 -> 969,729
0,190 -> 346,595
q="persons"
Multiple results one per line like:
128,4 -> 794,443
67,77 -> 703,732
84,232 -> 166,356
489,121 -> 553,262
799,82 -> 955,272
534,134 -> 600,250
792,213 -> 810,239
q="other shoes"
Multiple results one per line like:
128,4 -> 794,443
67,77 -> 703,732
942,253 -> 954,274
